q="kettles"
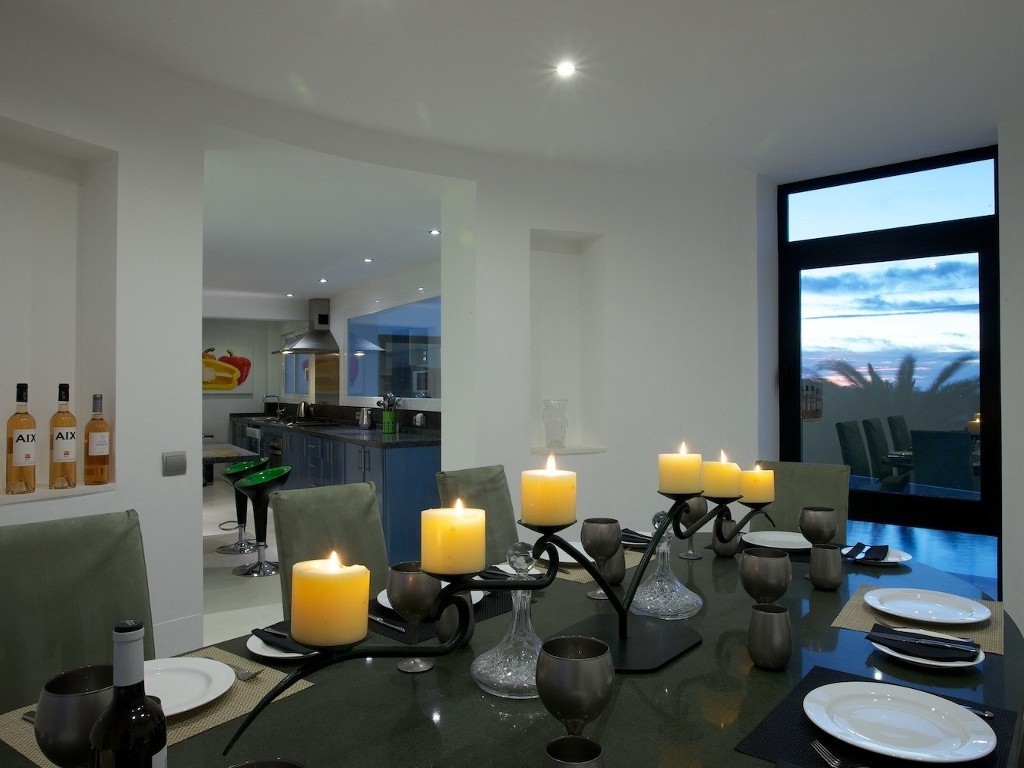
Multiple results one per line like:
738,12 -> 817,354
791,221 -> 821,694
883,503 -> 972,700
296,400 -> 311,417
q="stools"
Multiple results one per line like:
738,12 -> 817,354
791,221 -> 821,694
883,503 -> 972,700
216,457 -> 293,578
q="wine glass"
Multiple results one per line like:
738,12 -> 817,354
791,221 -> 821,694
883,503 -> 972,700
387,561 -> 437,672
581,517 -> 621,600
536,635 -> 615,764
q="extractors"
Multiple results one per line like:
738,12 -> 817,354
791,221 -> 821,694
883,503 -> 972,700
272,298 -> 340,354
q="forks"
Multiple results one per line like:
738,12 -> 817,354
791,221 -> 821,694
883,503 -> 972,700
874,618 -> 972,644
810,739 -> 859,768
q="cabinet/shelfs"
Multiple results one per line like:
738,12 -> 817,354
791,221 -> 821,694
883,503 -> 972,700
232,421 -> 441,566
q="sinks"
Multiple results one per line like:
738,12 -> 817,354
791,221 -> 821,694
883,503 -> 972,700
242,417 -> 273,421
253,421 -> 284,425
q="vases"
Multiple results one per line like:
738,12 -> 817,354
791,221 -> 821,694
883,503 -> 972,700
543,398 -> 567,449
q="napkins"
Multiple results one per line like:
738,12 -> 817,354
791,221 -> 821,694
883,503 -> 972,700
622,527 -> 652,544
480,565 -> 544,581
845,542 -> 889,561
1,533 -> 1024,768
250,618 -> 316,653
866,622 -> 982,661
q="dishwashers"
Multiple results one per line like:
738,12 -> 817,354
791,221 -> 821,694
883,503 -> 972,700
247,427 -> 261,454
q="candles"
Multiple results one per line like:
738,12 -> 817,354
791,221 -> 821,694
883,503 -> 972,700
658,440 -> 775,503
968,420 -> 980,435
421,499 -> 486,575
292,550 -> 369,646
521,453 -> 577,526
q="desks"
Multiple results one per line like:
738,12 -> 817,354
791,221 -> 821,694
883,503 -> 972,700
202,437 -> 260,487
881,454 -> 980,475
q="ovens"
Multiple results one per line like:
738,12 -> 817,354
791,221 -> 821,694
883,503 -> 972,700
268,436 -> 283,469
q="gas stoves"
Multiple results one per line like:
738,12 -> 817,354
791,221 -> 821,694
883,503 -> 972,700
269,414 -> 344,426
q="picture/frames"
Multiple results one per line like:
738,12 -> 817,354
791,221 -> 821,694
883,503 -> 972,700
293,353 -> 365,395
202,343 -> 254,394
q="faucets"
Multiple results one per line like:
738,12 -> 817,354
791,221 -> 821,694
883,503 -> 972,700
262,395 -> 280,421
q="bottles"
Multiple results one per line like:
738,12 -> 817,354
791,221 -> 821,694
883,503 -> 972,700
89,619 -> 168,768
471,542 -> 545,699
50,384 -> 77,489
6,383 -> 37,494
623,512 -> 702,620
84,394 -> 111,484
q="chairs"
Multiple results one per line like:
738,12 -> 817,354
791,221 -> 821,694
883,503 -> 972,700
749,460 -> 852,547
436,464 -> 520,569
836,415 -> 976,491
269,480 -> 391,622
0,508 -> 155,714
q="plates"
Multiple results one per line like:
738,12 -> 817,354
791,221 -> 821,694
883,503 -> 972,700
142,627 -> 324,718
741,531 -> 812,548
803,546 -> 996,762
538,542 -> 596,563
377,578 -> 485,609
620,530 -> 653,549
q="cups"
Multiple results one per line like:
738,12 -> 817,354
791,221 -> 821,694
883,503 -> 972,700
596,545 -> 626,585
34,664 -> 116,768
679,497 -> 843,671
